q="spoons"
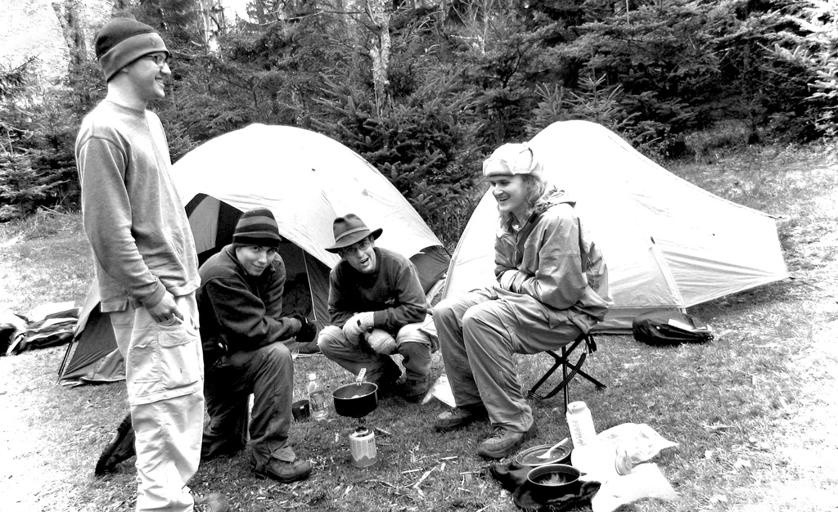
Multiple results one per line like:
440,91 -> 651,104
536,435 -> 570,460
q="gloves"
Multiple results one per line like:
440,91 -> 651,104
293,312 -> 317,344
342,311 -> 376,346
362,327 -> 397,354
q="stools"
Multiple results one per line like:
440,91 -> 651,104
525,328 -> 607,415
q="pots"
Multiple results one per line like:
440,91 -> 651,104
527,464 -> 587,502
331,367 -> 378,418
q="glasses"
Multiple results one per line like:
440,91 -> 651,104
340,241 -> 370,252
144,53 -> 168,64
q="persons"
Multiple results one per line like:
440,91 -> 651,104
430,142 -> 616,460
94,206 -> 317,485
72,14 -> 207,512
317,213 -> 439,402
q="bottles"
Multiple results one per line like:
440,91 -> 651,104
566,401 -> 595,447
308,374 -> 327,422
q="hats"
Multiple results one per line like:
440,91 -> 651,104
95,18 -> 170,84
481,142 -> 544,177
324,212 -> 384,253
233,207 -> 282,247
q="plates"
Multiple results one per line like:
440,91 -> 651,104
516,444 -> 572,467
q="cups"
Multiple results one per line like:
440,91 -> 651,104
291,400 -> 311,418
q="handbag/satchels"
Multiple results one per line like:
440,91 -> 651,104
632,310 -> 715,346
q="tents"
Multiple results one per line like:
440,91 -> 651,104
56,120 -> 449,390
444,118 -> 792,339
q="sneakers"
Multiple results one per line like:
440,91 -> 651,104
191,491 -> 230,512
479,422 -> 539,458
434,402 -> 487,431
250,451 -> 313,483
405,372 -> 431,401
94,413 -> 136,476
375,361 -> 402,400
